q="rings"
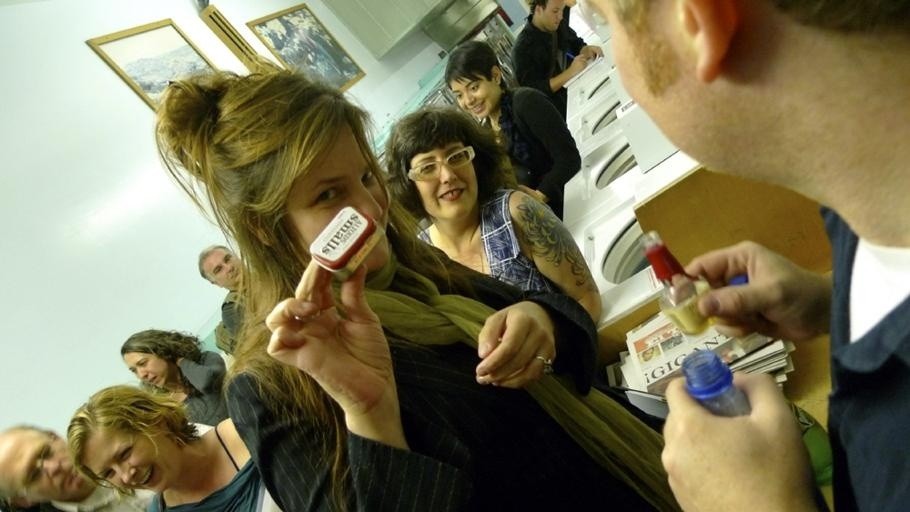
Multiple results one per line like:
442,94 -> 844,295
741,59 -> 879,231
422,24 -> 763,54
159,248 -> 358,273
535,356 -> 553,376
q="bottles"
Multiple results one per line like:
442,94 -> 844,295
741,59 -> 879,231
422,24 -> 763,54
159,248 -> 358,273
684,350 -> 752,418
645,239 -> 715,337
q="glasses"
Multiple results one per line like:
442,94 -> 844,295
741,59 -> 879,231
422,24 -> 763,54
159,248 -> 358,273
406,145 -> 476,182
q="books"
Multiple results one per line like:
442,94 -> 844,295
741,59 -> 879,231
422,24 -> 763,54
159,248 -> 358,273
606,307 -> 794,401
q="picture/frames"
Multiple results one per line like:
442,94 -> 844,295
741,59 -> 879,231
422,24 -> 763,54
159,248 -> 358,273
85,18 -> 218,112
246,3 -> 366,95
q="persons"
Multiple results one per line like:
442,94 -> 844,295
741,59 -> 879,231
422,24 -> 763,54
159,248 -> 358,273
642,348 -> 654,361
587,1 -> 910,512
511,0 -> 604,118
0,424 -> 221,512
69,388 -> 281,512
121,330 -> 228,426
446,43 -> 581,219
154,69 -> 682,512
386,108 -> 601,328
199,245 -> 252,335
